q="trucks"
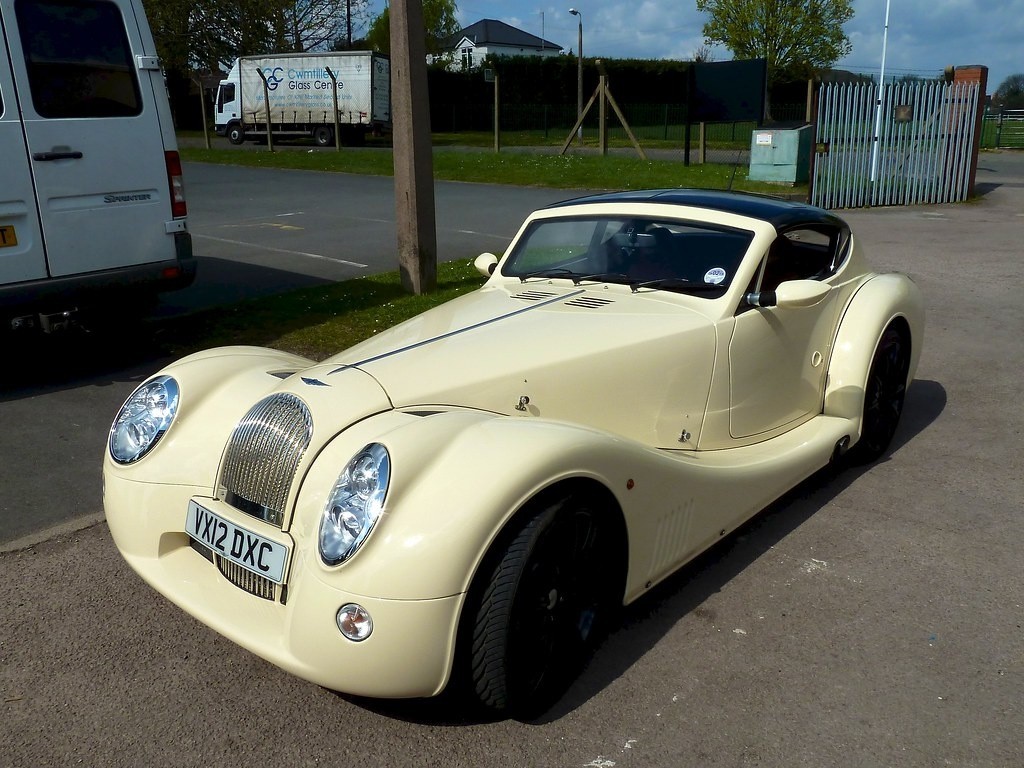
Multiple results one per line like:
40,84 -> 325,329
214,50 -> 390,146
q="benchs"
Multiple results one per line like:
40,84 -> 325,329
623,231 -> 830,286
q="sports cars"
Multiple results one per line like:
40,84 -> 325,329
100,186 -> 928,727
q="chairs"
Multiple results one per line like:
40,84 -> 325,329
747,235 -> 805,294
629,226 -> 682,280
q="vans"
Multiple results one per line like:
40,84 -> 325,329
1,0 -> 192,330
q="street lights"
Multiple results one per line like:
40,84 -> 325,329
568,8 -> 584,143
